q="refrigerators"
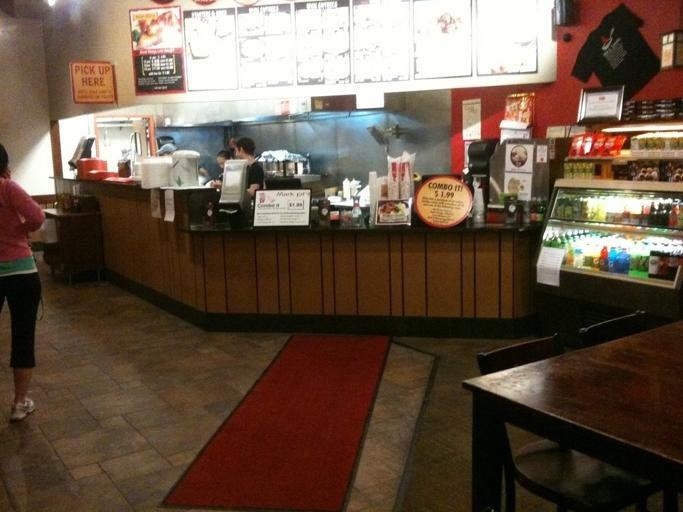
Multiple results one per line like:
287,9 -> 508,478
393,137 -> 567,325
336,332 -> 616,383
531,179 -> 682,320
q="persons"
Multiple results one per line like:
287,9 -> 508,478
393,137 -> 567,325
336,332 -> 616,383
210,150 -> 234,197
157,143 -> 176,156
0,140 -> 46,423
235,137 -> 264,200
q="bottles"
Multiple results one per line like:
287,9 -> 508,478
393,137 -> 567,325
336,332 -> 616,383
543,196 -> 683,279
351,195 -> 364,228
530,196 -> 547,226
311,199 -> 319,224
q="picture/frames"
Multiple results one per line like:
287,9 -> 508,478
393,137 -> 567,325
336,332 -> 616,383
576,83 -> 626,124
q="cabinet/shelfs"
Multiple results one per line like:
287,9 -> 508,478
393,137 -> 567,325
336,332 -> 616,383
41,207 -> 105,286
534,121 -> 683,324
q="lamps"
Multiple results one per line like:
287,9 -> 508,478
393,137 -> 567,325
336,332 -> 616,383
660,30 -> 683,71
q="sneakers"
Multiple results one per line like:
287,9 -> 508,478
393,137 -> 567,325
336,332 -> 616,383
10,396 -> 36,422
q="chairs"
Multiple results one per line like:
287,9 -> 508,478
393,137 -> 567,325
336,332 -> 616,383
476,333 -> 664,512
576,309 -> 650,349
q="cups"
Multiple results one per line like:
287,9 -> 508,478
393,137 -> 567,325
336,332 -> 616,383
473,187 -> 485,214
369,159 -> 415,217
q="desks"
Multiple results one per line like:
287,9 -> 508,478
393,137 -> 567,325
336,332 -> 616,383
461,320 -> 683,512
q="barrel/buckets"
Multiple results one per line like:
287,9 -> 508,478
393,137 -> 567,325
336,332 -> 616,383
171,156 -> 198,187
